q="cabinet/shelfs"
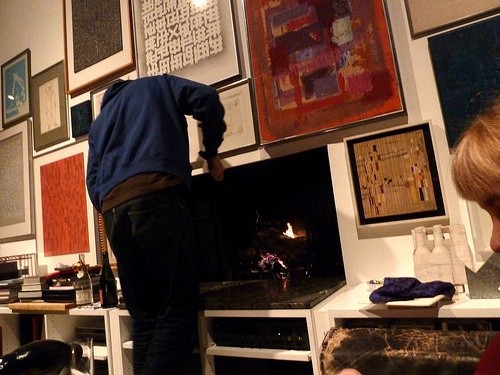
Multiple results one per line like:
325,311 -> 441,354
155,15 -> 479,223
0,283 -> 500,375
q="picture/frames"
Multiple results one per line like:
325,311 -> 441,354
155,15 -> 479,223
343,120 -> 450,228
242,0 -> 407,146
32,136 -> 99,274
132,0 -> 240,89
30,60 -> 69,150
404,0 -> 500,39
0,120 -> 36,244
63,0 -> 135,94
0,49 -> 32,127
216,78 -> 258,156
90,79 -> 122,122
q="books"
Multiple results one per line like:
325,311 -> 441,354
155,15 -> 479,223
0,274 -> 47,304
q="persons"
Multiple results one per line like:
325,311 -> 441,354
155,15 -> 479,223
450,97 -> 500,375
85,72 -> 226,375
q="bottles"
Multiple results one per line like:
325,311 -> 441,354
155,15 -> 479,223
99,251 -> 118,309
75,254 -> 93,307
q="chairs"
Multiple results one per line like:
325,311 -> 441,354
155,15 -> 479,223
0,339 -> 83,374
